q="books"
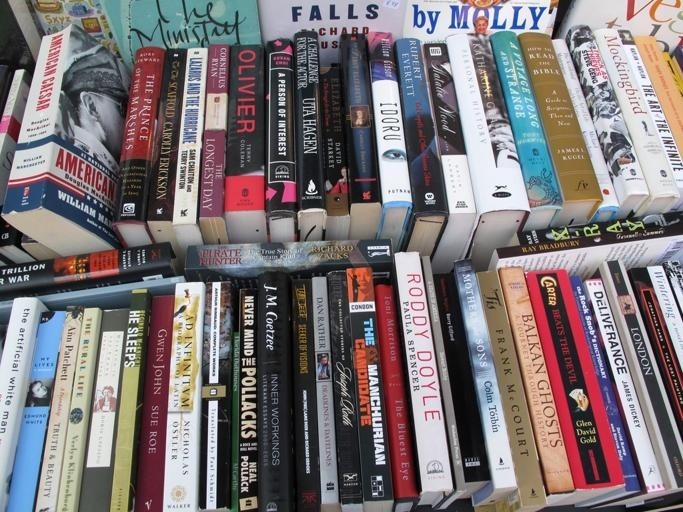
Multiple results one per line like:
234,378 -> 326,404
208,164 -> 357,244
0,0 -> 683,512
